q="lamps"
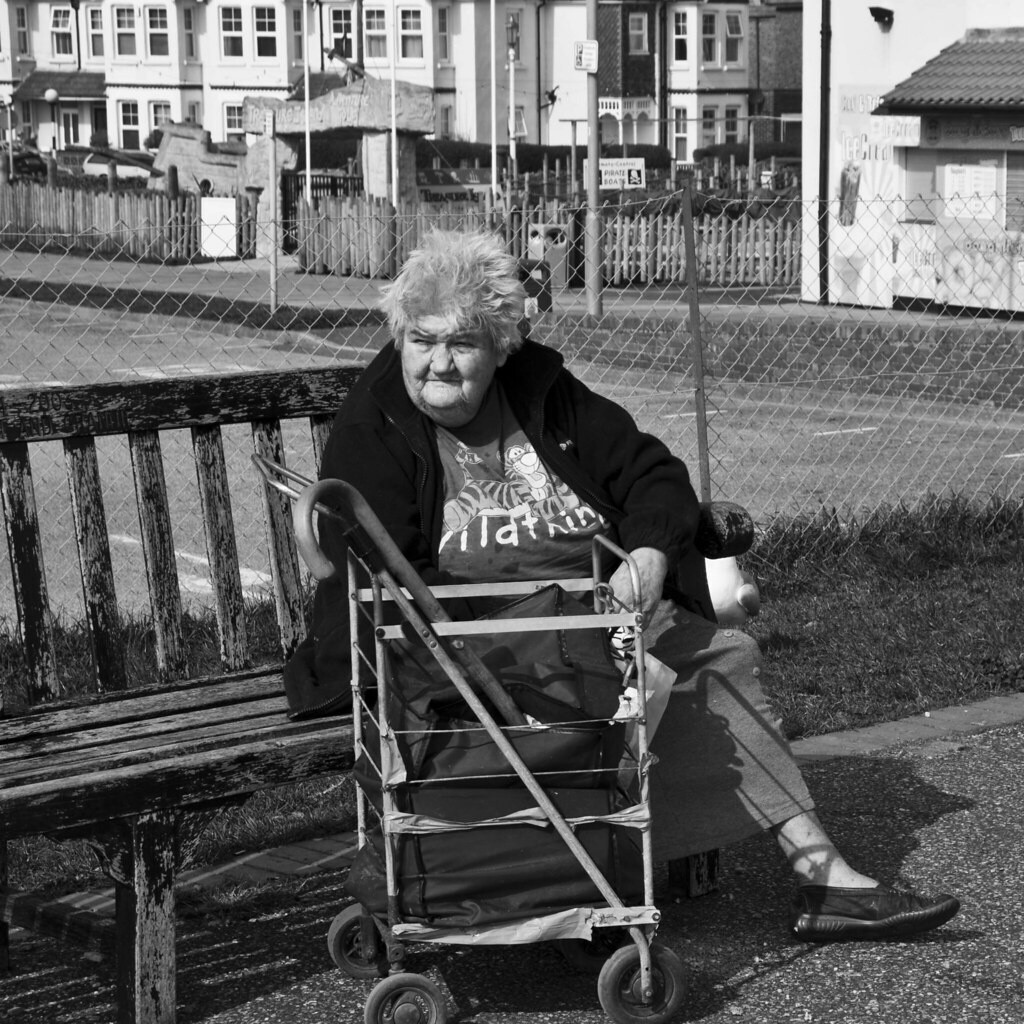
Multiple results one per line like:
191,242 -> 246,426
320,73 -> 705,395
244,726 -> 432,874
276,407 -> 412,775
870,7 -> 893,24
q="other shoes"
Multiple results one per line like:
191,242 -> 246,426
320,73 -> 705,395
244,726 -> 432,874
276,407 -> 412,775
580,926 -> 626,961
789,881 -> 960,941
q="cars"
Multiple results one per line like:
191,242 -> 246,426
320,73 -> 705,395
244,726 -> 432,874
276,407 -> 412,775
0,148 -> 156,179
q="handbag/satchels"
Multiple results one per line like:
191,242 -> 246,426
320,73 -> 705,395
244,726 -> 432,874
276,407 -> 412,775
345,583 -> 670,925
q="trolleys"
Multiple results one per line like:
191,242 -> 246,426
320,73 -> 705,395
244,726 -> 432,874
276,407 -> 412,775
251,453 -> 688,1023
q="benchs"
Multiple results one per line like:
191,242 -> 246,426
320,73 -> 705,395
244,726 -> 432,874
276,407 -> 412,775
1,365 -> 760,1024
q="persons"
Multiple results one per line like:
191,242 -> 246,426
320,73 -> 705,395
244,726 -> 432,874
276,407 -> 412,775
282,230 -> 961,942
